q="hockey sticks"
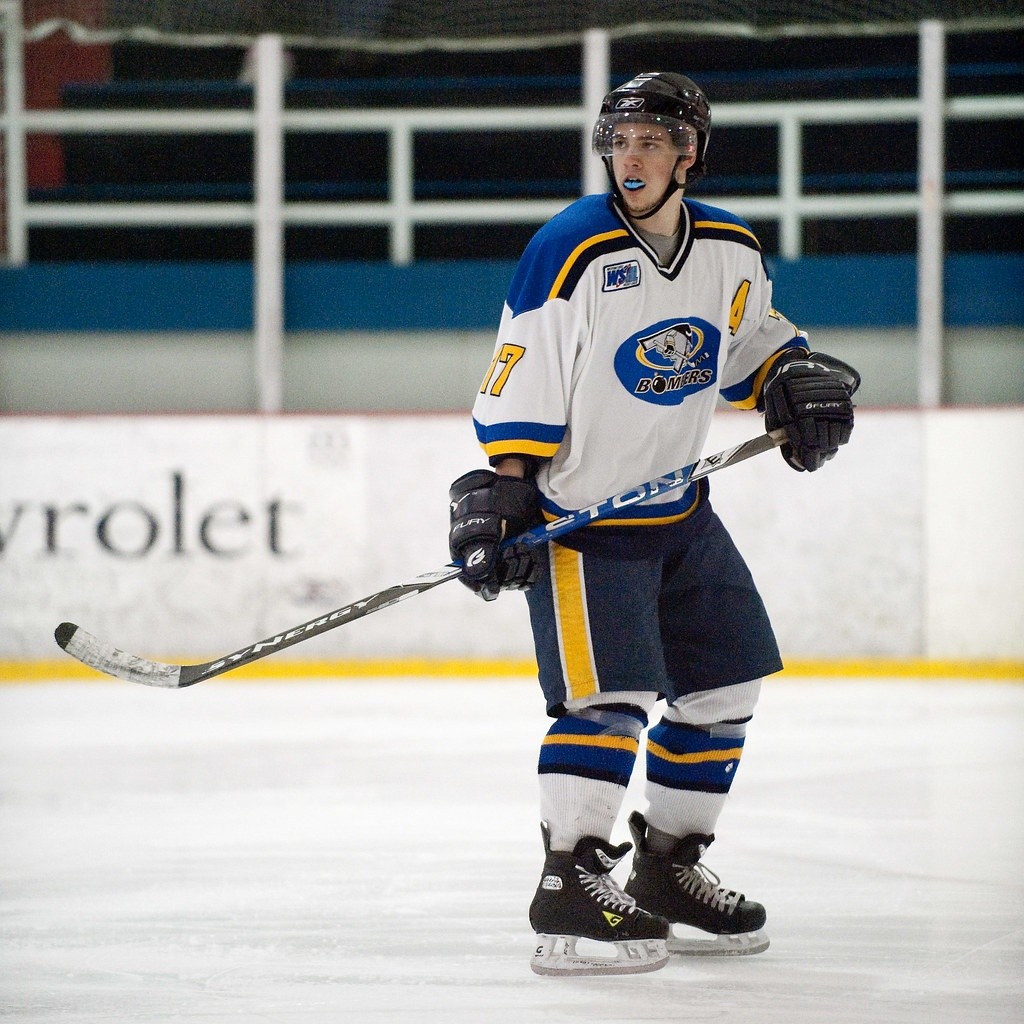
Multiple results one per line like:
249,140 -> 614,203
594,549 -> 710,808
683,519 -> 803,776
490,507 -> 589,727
54,428 -> 784,694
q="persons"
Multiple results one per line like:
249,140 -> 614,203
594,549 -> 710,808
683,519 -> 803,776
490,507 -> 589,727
448,71 -> 861,977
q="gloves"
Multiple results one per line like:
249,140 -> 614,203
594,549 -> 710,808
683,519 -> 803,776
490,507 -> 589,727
448,471 -> 543,601
764,348 -> 861,472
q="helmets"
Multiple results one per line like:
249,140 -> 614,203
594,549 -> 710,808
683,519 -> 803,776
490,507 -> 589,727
592,73 -> 712,172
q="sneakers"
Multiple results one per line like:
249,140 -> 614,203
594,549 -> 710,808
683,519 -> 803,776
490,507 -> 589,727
623,811 -> 770,955
527,823 -> 668,976
